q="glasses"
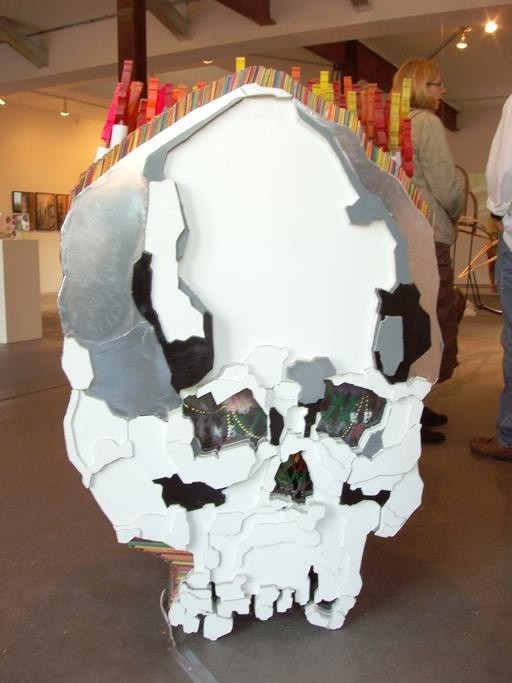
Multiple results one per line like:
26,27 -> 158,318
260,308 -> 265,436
425,79 -> 445,87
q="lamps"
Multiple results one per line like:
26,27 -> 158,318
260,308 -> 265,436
456,26 -> 468,50
60,97 -> 70,117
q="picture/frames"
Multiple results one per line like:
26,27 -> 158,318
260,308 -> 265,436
12,191 -> 70,231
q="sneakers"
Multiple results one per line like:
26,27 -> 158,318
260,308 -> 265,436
466,432 -> 512,462
420,404 -> 448,443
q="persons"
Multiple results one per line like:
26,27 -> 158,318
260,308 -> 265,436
469,95 -> 512,459
387,58 -> 465,444
